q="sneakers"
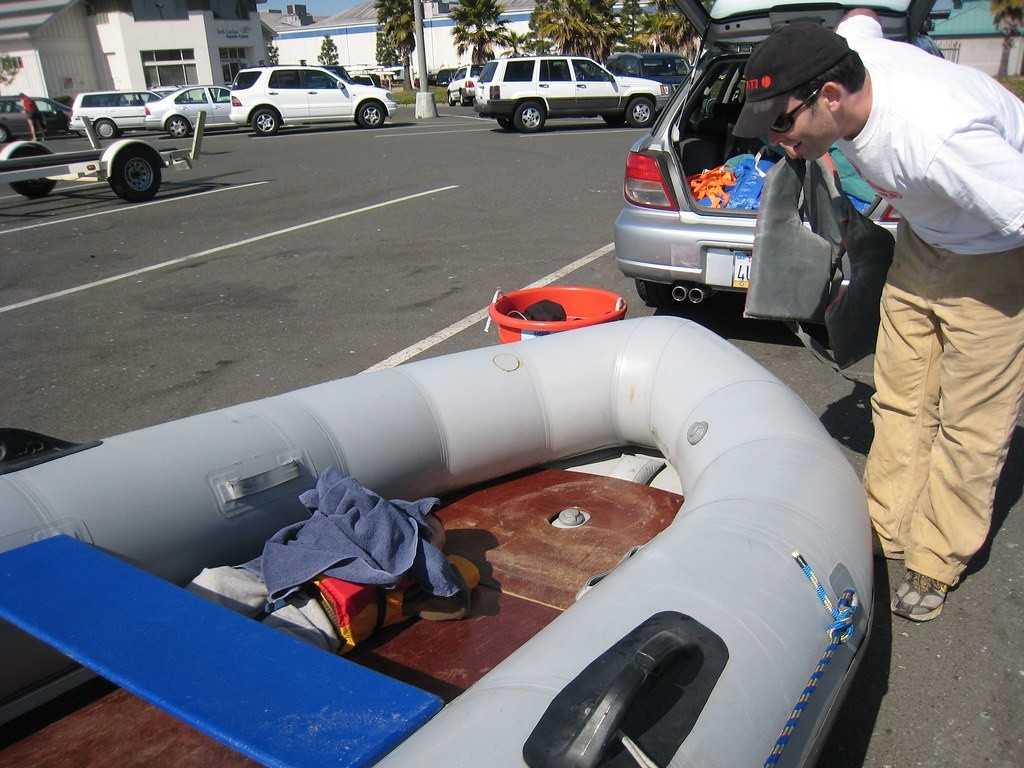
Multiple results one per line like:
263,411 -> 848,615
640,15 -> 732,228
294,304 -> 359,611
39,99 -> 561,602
890,569 -> 949,621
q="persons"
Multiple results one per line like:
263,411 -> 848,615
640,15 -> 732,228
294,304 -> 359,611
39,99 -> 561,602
19,93 -> 46,142
731,7 -> 1024,622
185,512 -> 470,656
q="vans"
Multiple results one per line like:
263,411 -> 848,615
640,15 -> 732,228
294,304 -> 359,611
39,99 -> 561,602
69,90 -> 164,139
0,96 -> 88,143
228,65 -> 397,136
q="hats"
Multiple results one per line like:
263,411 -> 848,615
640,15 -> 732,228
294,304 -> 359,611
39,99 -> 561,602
731,24 -> 848,137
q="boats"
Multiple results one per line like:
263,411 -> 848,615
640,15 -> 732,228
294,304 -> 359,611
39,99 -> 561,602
0,314 -> 876,767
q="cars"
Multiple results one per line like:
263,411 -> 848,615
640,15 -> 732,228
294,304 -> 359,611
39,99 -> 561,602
414,78 -> 420,87
601,53 -> 695,123
614,1 -> 948,302
428,74 -> 438,85
447,64 -> 487,107
438,68 -> 457,87
473,53 -> 669,133
305,65 -> 360,88
217,85 -> 233,102
143,85 -> 239,138
344,65 -> 417,91
146,86 -> 193,104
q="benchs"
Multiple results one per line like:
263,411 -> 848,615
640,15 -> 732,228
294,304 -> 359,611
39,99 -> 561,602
0,533 -> 443,768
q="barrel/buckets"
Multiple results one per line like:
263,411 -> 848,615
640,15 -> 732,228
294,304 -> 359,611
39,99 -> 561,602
490,286 -> 627,345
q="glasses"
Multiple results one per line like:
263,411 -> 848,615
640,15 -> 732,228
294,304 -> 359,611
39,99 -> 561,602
769,85 -> 823,132
